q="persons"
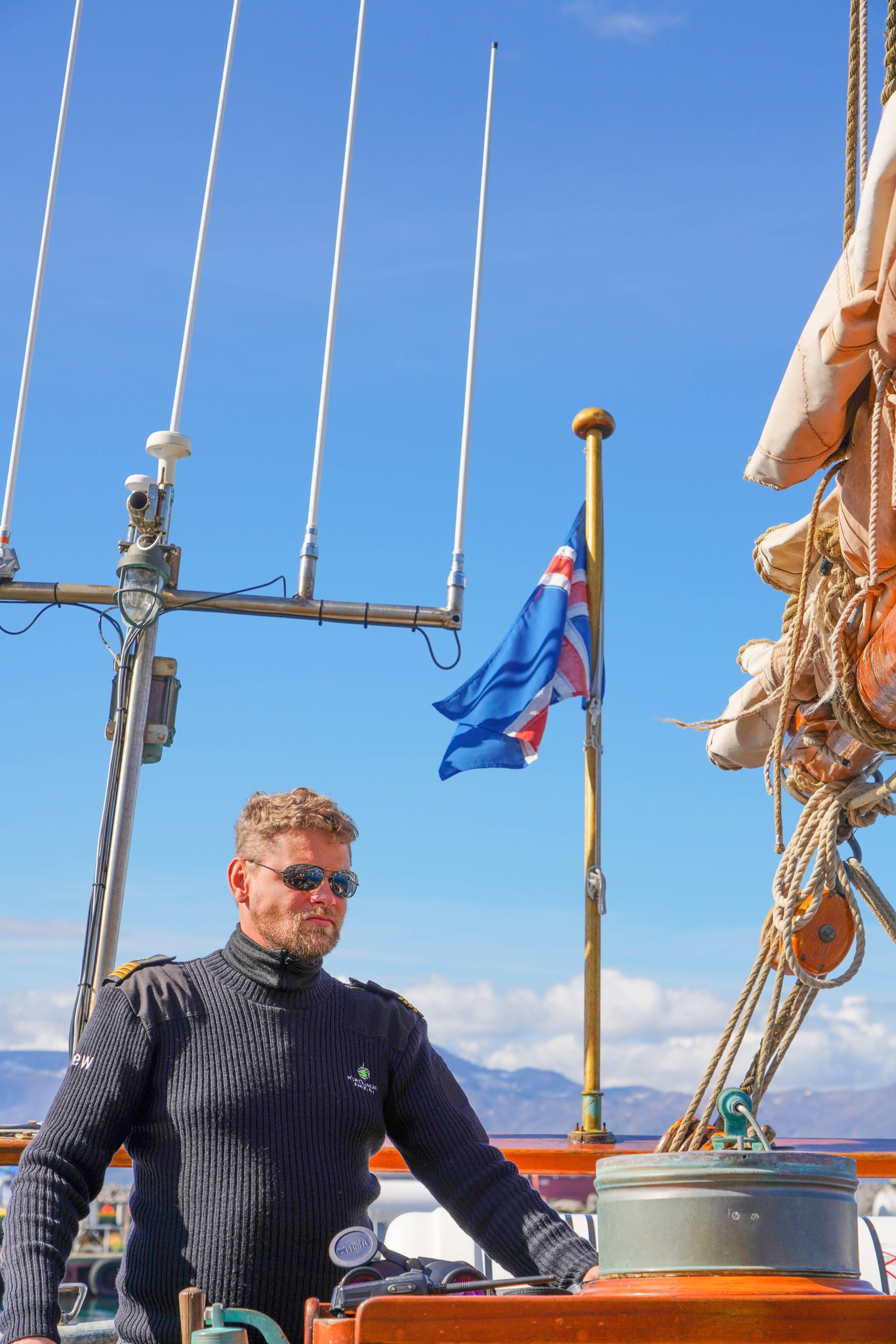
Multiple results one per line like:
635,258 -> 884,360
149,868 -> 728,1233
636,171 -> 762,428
0,785 -> 601,1344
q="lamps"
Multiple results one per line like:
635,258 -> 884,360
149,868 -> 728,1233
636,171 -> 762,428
116,543 -> 171,627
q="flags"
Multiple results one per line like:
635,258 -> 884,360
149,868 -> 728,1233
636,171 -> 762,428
431,496 -> 606,781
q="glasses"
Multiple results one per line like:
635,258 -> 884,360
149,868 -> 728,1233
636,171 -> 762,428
243,859 -> 359,898
